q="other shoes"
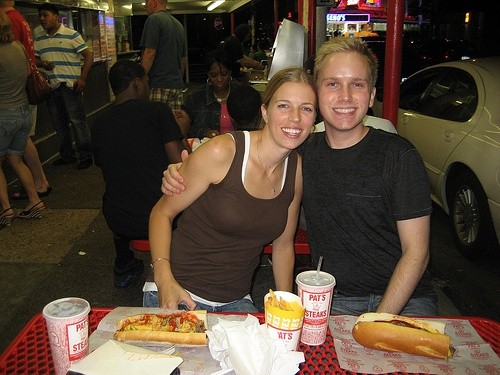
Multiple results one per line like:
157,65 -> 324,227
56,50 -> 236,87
76,158 -> 93,169
53,155 -> 77,164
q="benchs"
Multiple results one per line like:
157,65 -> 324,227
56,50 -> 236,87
131,227 -> 311,261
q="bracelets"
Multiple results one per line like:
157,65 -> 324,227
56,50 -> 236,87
152,257 -> 171,266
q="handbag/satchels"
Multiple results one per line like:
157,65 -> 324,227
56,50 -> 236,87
26,69 -> 52,104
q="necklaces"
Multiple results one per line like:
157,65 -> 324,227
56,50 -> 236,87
257,131 -> 279,193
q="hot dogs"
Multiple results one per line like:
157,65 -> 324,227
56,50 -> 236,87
352,312 -> 451,360
114,310 -> 209,346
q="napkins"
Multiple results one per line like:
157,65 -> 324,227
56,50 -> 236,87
204,314 -> 306,375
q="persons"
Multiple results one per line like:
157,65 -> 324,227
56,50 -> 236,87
181,50 -> 261,138
161,37 -> 438,317
90,60 -> 191,288
32,5 -> 94,169
214,24 -> 261,69
142,68 -> 317,314
139,0 -> 186,110
0,0 -> 53,226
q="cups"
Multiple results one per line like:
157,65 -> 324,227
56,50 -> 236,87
295,271 -> 337,345
43,296 -> 91,375
264,290 -> 305,350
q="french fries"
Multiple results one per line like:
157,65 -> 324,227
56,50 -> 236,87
266,288 -> 293,312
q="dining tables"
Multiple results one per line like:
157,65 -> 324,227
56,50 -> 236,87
0,306 -> 500,375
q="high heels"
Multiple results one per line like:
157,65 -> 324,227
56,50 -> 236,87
17,200 -> 45,219
0,207 -> 14,226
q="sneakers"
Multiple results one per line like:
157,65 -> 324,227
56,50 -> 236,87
114,259 -> 144,287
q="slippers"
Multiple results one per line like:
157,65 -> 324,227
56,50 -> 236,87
10,184 -> 52,199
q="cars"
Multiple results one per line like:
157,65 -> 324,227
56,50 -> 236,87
366,56 -> 500,262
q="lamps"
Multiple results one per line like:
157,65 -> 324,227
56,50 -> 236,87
207,0 -> 225,12
